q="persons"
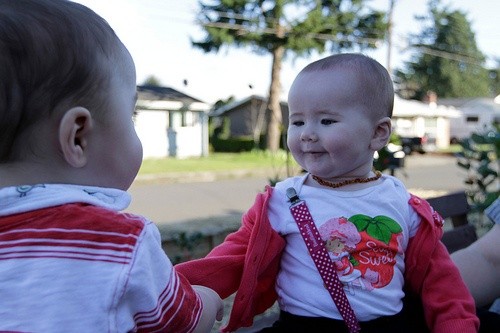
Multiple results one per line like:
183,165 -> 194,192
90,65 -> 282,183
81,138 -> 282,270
449,194 -> 500,333
170,52 -> 482,333
0,0 -> 225,333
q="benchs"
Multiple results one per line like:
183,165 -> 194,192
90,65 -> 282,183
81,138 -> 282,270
423,190 -> 499,333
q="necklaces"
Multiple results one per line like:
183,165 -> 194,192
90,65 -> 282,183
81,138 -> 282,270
312,170 -> 383,188
